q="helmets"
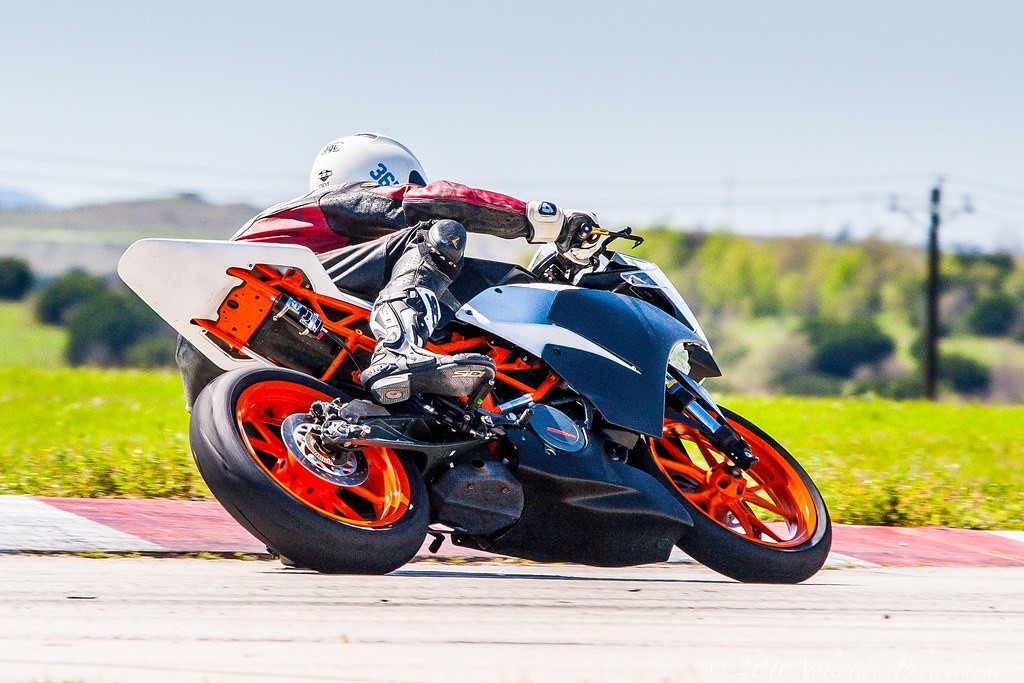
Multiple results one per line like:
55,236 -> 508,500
310,132 -> 428,192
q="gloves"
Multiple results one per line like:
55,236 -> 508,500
526,200 -> 602,266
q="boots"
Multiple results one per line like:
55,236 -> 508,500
361,284 -> 495,405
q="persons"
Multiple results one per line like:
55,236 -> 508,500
175,134 -> 601,405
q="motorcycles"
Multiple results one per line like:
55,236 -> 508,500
117,207 -> 835,587
